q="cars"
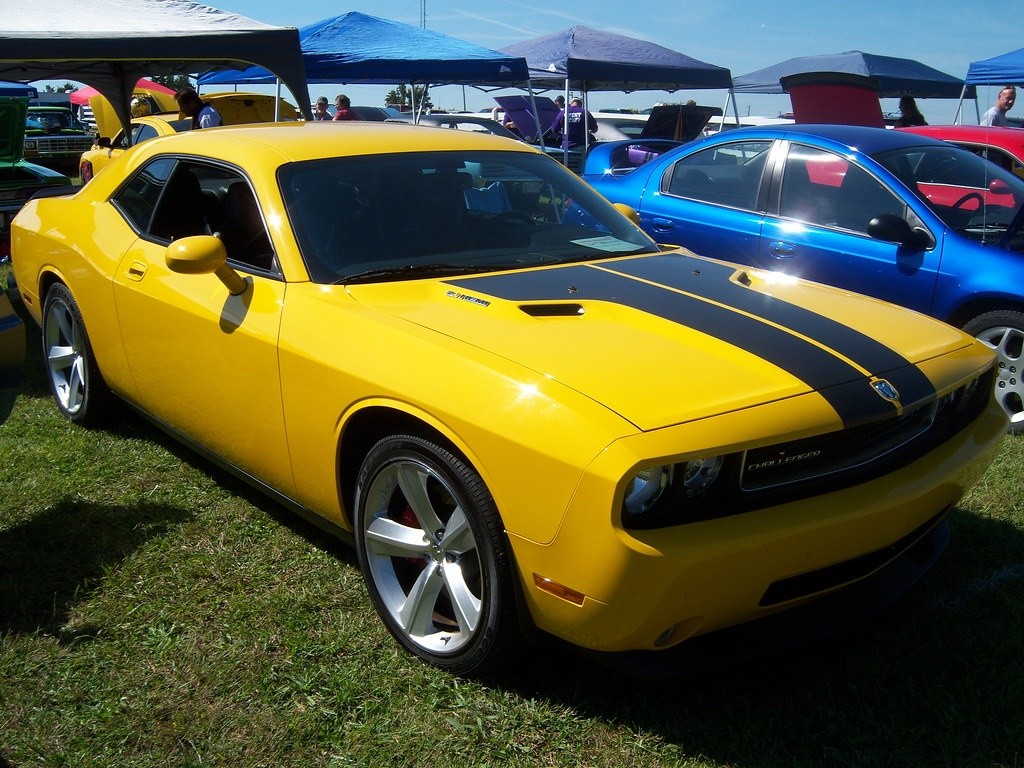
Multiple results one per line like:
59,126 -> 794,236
22,106 -> 95,161
578,122 -> 1024,439
805,124 -> 1024,211
79,113 -> 193,186
1,159 -> 73,213
384,112 -> 583,177
7,121 -> 1013,679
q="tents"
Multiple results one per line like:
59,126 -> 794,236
714,49 -> 981,163
0,0 -> 314,140
70,78 -> 179,129
952,45 -> 1024,127
416,24 -> 739,150
196,11 -> 546,154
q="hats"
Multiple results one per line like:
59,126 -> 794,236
555,95 -> 565,102
570,96 -> 583,104
316,96 -> 329,105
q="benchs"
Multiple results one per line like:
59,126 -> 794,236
674,165 -> 761,213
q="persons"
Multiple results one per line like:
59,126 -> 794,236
502,95 -> 598,149
894,96 -> 930,127
174,87 -> 223,128
980,85 -> 1016,126
332,95 -> 362,121
312,96 -> 333,120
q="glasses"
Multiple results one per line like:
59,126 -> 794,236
999,85 -> 1015,94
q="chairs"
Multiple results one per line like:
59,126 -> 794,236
782,155 -> 826,228
836,164 -> 883,235
